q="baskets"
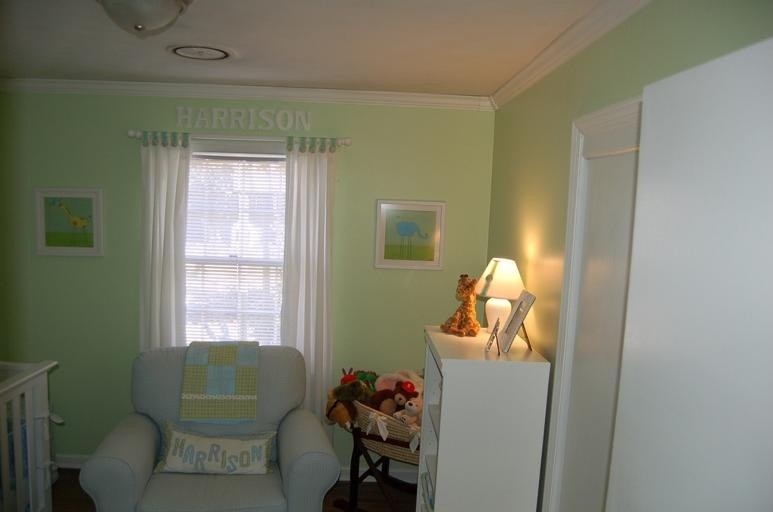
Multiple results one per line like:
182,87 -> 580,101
353,401 -> 419,465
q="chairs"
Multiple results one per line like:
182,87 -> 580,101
77,345 -> 341,510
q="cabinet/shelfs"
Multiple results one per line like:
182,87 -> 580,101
416,322 -> 550,511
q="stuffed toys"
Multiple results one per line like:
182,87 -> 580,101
324,364 -> 422,433
440,274 -> 480,337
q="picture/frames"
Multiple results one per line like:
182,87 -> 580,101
485,289 -> 535,354
374,199 -> 447,271
34,185 -> 103,257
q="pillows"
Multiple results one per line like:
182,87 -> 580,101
153,415 -> 279,475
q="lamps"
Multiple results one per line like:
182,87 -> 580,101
97,0 -> 186,38
473,256 -> 526,332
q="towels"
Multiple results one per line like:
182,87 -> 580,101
179,340 -> 259,418
